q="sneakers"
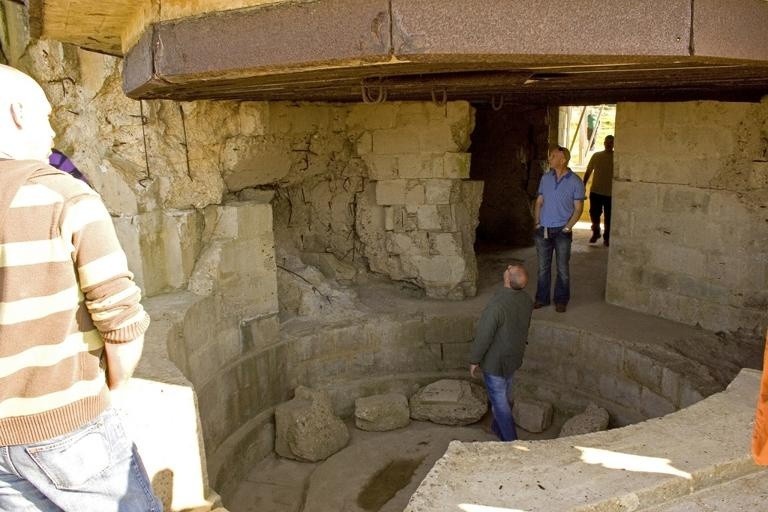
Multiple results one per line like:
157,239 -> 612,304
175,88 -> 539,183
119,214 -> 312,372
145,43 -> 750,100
604,238 -> 608,246
555,303 -> 566,313
590,233 -> 601,243
533,298 -> 551,309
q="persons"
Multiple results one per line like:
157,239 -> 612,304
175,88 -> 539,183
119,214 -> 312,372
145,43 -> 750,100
532,144 -> 588,313
1,63 -> 169,512
588,108 -> 599,151
469,263 -> 533,442
582,135 -> 615,248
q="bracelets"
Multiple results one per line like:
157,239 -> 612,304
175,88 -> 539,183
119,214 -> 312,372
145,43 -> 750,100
535,222 -> 540,226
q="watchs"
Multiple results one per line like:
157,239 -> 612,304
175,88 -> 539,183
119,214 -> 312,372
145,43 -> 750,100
564,225 -> 572,233
